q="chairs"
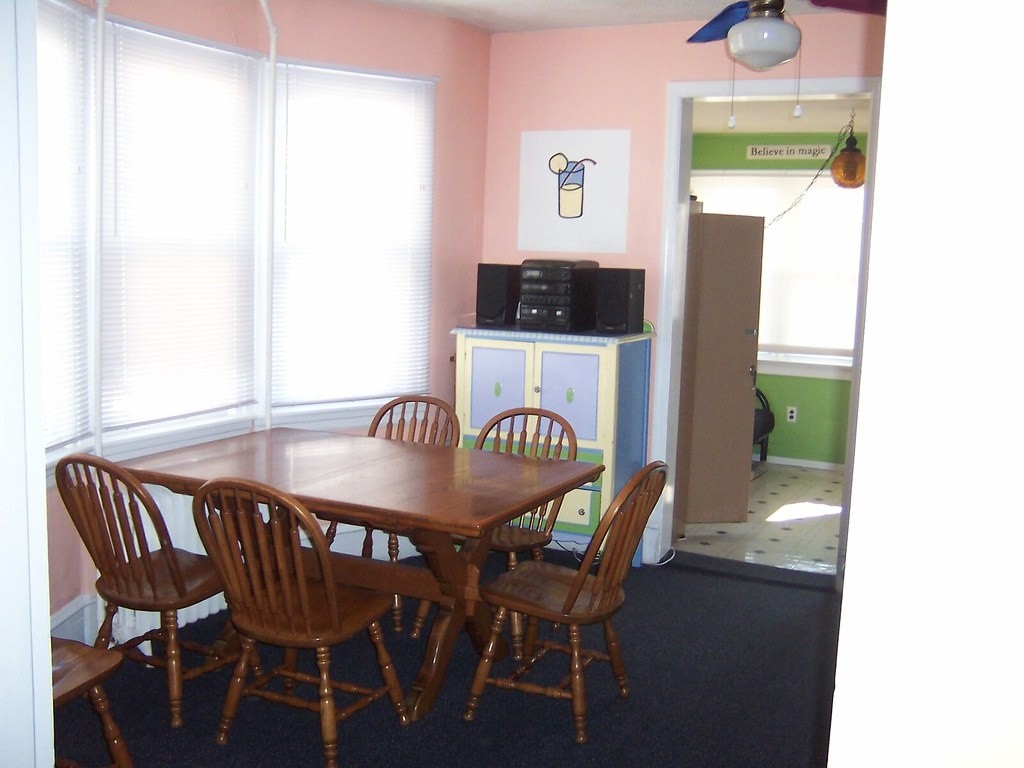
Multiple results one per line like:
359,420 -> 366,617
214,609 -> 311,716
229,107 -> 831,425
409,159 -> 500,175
411,407 -> 577,659
51,634 -> 133,768
323,393 -> 463,632
191,477 -> 412,768
462,460 -> 669,742
748,388 -> 775,480
55,451 -> 267,725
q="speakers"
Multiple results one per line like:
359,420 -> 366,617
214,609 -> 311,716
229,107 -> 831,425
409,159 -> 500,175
475,263 -> 521,330
595,267 -> 647,335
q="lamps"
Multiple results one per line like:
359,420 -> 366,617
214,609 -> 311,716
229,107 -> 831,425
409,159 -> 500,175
831,121 -> 866,189
726,0 -> 804,129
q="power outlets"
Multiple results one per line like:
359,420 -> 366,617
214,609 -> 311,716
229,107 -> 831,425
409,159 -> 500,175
787,406 -> 797,421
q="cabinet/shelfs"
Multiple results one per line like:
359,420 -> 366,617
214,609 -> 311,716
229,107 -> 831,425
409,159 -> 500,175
450,327 -> 658,570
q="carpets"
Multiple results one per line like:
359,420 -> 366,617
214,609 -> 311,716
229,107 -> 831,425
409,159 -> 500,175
56,547 -> 836,767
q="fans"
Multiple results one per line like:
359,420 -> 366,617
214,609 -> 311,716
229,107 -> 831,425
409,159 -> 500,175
686,1 -> 887,45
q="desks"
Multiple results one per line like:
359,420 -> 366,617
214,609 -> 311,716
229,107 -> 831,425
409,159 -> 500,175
110,425 -> 606,724
